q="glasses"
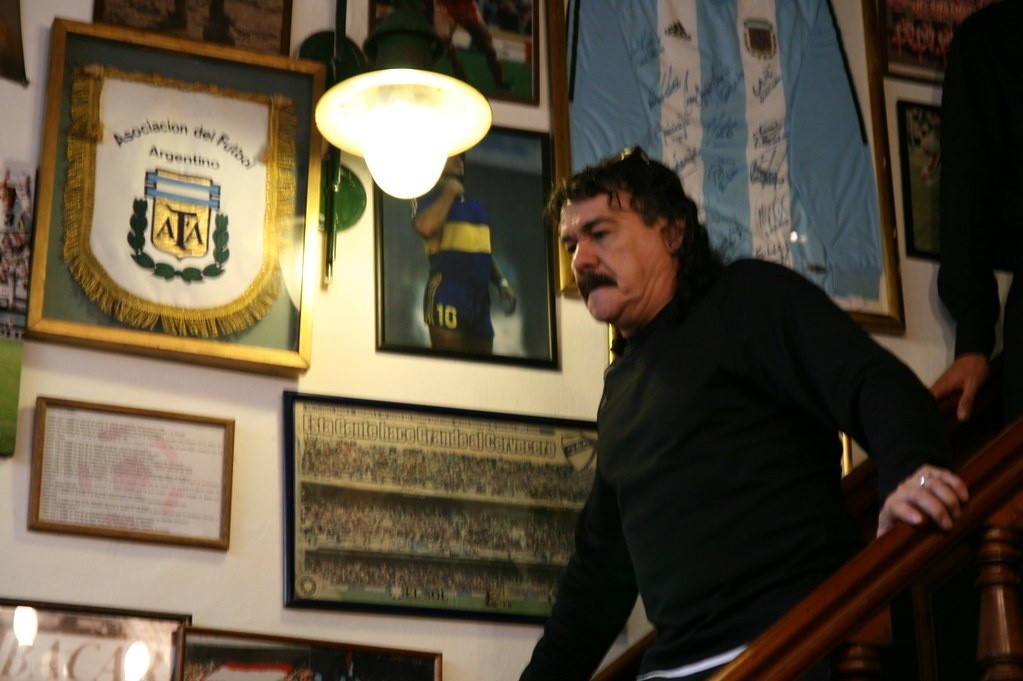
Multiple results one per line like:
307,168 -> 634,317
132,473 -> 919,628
609,143 -> 651,167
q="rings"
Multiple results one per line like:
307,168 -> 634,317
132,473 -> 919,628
920,472 -> 932,489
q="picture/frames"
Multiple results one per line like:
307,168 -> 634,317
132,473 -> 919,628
544,1 -> 909,337
173,624 -> 444,681
367,129 -> 563,373
896,97 -> 942,263
283,389 -> 599,624
0,597 -> 193,681
25,16 -> 329,376
368,0 -> 540,107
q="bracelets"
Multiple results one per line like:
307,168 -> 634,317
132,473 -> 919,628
497,278 -> 510,288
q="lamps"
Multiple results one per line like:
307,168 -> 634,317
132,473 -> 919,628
313,1 -> 493,203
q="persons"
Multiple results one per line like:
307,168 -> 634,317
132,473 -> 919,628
522,144 -> 971,680
926,0 -> 1023,458
408,152 -> 517,355
155,0 -> 236,44
433,0 -> 513,88
